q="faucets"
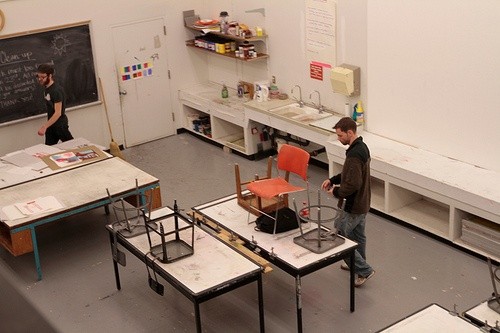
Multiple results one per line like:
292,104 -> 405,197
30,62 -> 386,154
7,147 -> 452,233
290,84 -> 302,108
309,90 -> 321,114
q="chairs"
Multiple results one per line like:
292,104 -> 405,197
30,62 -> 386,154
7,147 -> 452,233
142,199 -> 196,296
247,143 -> 311,240
292,181 -> 352,268
234,155 -> 290,216
105,179 -> 158,266
487,256 -> 500,314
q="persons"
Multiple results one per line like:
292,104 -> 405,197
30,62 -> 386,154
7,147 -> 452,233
320,117 -> 375,286
36,63 -> 74,146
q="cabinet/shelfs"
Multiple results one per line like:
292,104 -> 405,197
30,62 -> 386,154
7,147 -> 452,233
180,90 -> 272,155
185,26 -> 268,63
0,151 -> 161,282
326,130 -> 500,264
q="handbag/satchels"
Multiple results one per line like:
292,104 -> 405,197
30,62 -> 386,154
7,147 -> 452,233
256,207 -> 300,234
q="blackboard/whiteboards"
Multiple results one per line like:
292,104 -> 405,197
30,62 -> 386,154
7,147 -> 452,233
0,19 -> 104,128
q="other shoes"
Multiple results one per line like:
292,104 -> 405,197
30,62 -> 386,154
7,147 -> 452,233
341,264 -> 350,270
354,269 -> 375,287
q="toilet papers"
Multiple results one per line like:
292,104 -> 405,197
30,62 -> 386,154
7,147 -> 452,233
344,104 -> 350,117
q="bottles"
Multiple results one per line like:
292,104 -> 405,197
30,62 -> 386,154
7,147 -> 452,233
194,20 -> 263,59
222,85 -> 228,99
270,75 -> 278,95
301,201 -> 308,224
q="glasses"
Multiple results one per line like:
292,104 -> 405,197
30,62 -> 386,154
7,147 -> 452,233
36,76 -> 48,80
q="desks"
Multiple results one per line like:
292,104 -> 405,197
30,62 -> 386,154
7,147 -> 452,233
374,300 -> 500,333
105,205 -> 264,333
192,189 -> 359,333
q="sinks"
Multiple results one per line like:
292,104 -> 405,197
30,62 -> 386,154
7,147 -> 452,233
295,111 -> 332,126
267,102 -> 313,120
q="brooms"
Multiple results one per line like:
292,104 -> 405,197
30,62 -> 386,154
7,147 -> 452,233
98,77 -> 127,161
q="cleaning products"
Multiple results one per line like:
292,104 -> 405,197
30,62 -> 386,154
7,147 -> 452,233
352,100 -> 364,123
